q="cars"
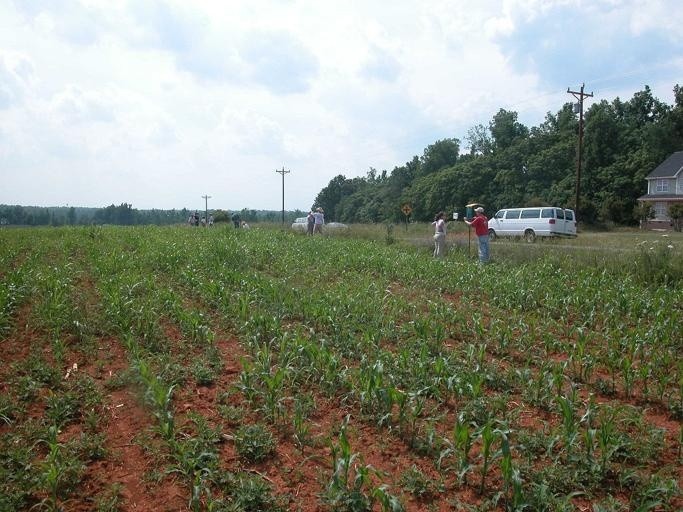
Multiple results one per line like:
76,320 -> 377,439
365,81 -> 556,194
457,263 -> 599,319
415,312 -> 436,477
292,217 -> 308,231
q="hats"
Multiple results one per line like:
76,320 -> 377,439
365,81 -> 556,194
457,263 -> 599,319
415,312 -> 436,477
474,207 -> 484,213
316,207 -> 324,212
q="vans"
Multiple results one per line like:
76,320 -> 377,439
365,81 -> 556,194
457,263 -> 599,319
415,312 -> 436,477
488,206 -> 578,243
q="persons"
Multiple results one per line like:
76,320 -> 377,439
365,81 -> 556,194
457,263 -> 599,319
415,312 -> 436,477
241,221 -> 250,229
207,213 -> 214,226
187,214 -> 194,226
310,207 -> 324,235
306,210 -> 315,237
230,209 -> 241,229
462,206 -> 489,262
430,210 -> 447,258
200,216 -> 205,227
